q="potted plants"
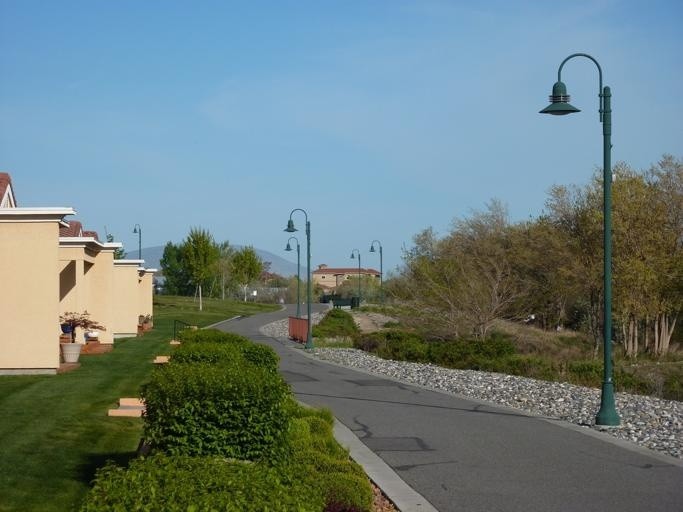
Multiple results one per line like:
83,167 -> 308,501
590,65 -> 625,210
59,309 -> 107,363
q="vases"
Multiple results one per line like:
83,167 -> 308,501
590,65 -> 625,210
60,323 -> 100,339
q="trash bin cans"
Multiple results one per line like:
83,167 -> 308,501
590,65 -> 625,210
351,297 -> 360,309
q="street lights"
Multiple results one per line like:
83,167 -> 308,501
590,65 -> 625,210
133,222 -> 142,259
349,248 -> 361,296
368,238 -> 383,304
282,208 -> 313,349
536,52 -> 625,427
284,236 -> 302,316
